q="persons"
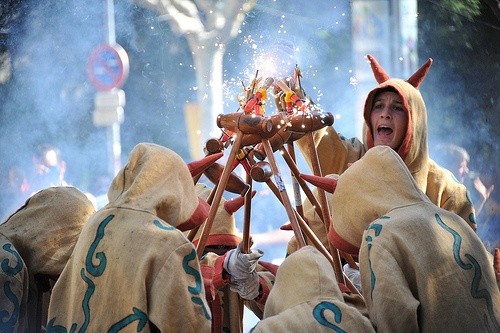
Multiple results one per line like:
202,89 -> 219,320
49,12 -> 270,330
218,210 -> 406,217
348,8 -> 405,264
270,54 -> 499,298
0,186 -> 97,333
247,244 -> 377,332
279,186 -> 363,303
44,142 -> 213,333
174,181 -> 282,332
423,144 -> 499,261
0,139 -> 115,236
329,143 -> 500,333
